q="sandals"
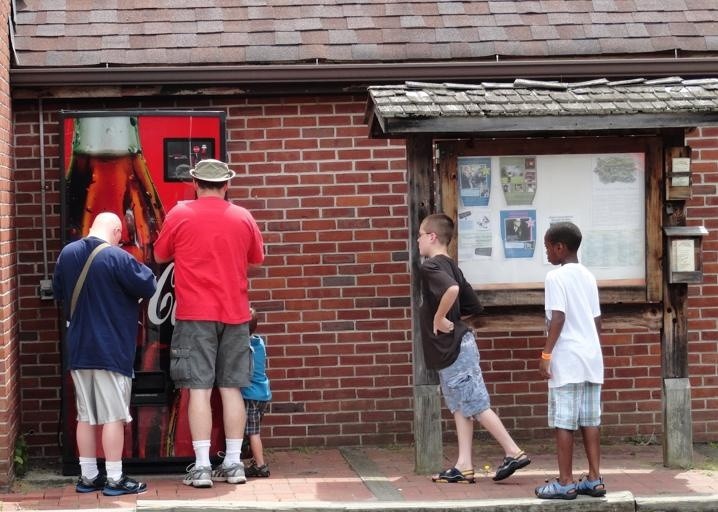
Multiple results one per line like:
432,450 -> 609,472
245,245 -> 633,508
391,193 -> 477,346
535,479 -> 576,499
493,451 -> 530,481
245,456 -> 271,477
575,473 -> 606,496
432,466 -> 475,484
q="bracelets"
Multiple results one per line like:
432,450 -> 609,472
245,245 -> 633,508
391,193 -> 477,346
539,350 -> 553,361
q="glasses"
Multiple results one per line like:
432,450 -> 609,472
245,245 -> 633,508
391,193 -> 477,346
416,232 -> 430,238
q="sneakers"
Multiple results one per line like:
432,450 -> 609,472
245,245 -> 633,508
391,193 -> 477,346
102,474 -> 148,496
182,464 -> 213,487
76,474 -> 106,493
212,462 -> 246,484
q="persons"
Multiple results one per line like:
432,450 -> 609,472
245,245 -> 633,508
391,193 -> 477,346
155,158 -> 266,488
477,215 -> 492,228
52,211 -> 158,497
534,222 -> 606,499
416,213 -> 531,484
237,306 -> 273,477
459,158 -> 537,198
505,217 -> 529,242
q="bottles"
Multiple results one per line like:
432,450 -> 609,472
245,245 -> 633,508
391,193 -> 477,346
62,115 -> 184,460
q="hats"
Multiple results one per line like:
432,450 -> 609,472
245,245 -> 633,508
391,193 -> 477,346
189,158 -> 236,183
